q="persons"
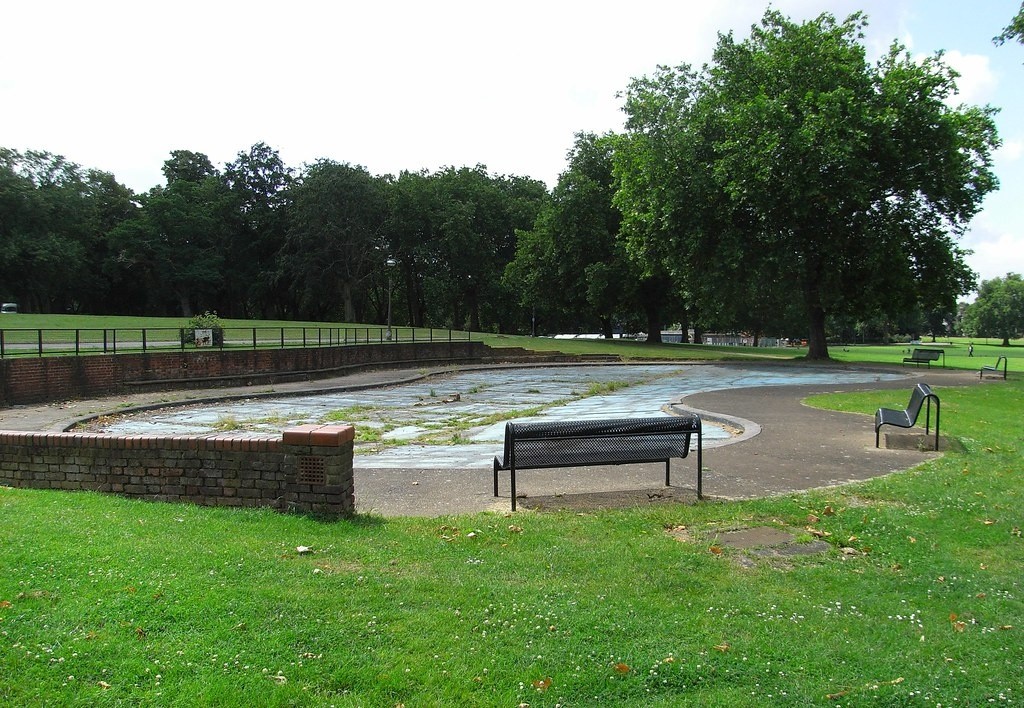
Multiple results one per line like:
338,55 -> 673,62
796,339 -> 800,349
688,334 -> 692,343
743,338 -> 747,346
968,345 -> 974,357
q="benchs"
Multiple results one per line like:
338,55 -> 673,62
903,349 -> 945,369
875,383 -> 940,450
980,354 -> 1007,380
493,413 -> 702,512
